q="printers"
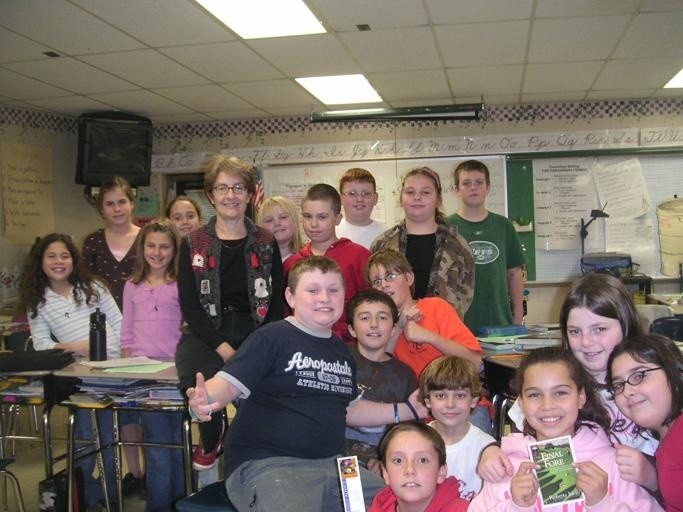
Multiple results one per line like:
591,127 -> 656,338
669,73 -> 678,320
580,251 -> 632,279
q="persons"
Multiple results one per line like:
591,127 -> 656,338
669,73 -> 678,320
476,273 -> 660,496
463,346 -> 665,512
13,155 -> 529,511
604,333 -> 683,512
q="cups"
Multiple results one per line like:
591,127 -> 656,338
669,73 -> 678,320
655,196 -> 683,278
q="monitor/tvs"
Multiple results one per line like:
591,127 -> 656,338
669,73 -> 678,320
74,111 -> 154,188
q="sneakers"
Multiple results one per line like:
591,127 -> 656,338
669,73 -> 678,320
121,472 -> 147,499
191,434 -> 224,471
87,498 -> 120,512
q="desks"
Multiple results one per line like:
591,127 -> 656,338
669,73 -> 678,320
481,324 -> 565,440
0,320 -> 29,352
0,382 -> 196,512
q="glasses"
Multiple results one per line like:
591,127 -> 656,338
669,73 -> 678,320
610,365 -> 665,395
214,183 -> 249,194
342,191 -> 373,199
373,272 -> 401,286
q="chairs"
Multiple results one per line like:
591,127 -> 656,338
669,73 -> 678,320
175,480 -> 236,511
0,336 -> 46,456
1,459 -> 26,512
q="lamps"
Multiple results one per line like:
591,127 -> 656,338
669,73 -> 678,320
579,208 -> 609,257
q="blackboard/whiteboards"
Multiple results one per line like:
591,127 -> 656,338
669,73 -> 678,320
506,146 -> 683,287
260,155 -> 509,248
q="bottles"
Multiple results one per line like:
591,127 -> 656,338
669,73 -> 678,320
88,306 -> 108,362
